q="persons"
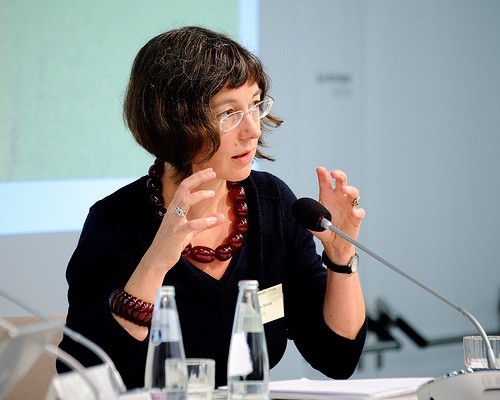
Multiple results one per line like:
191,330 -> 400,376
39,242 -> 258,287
56,26 -> 367,392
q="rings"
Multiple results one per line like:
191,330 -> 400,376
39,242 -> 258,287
175,206 -> 187,216
350,198 -> 359,207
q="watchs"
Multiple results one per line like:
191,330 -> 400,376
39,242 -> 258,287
322,250 -> 359,274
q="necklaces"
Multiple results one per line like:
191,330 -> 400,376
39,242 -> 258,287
147,158 -> 248,263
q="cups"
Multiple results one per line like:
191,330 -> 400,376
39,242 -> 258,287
463,335 -> 500,372
164,358 -> 216,400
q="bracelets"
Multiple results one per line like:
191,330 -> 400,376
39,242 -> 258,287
108,289 -> 154,326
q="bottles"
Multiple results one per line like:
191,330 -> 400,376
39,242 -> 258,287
225,280 -> 270,400
144,286 -> 189,400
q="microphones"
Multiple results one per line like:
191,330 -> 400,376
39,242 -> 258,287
291,197 -> 500,399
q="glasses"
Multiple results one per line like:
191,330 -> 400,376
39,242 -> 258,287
216,94 -> 274,132
